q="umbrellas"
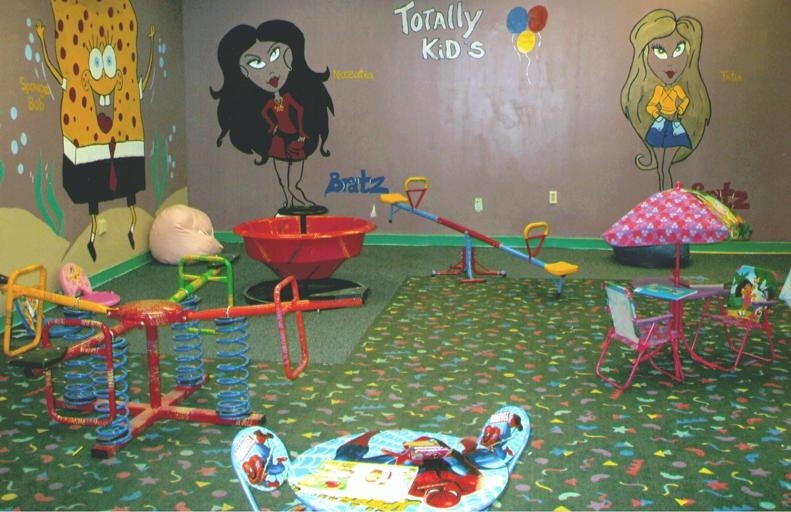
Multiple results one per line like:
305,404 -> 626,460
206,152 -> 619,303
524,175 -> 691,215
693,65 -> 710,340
599,177 -> 756,290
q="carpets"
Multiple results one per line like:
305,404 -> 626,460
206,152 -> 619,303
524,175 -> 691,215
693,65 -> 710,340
42,246 -> 430,367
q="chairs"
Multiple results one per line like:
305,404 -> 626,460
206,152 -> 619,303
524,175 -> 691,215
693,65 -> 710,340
596,265 -> 777,391
